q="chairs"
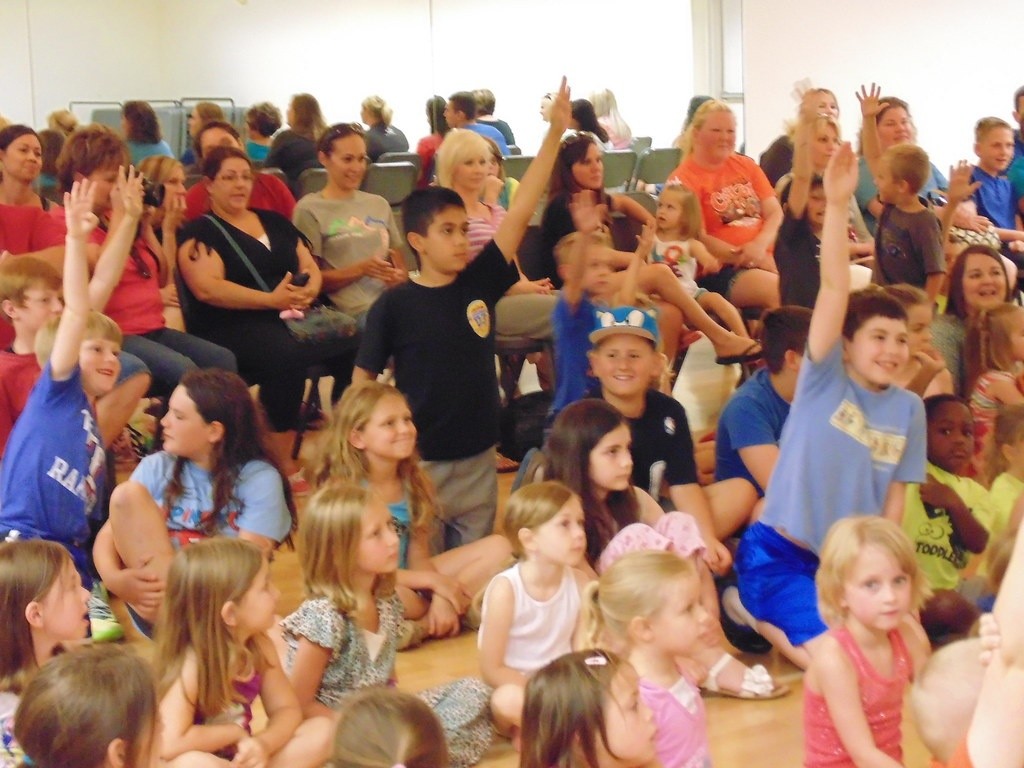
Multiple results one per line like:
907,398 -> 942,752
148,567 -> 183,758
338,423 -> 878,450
173,137 -> 683,474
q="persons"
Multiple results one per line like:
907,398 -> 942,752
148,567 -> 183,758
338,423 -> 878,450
801,517 -> 929,768
541,399 -> 791,700
518,648 -> 660,767
472,480 -> 596,749
718,142 -> 930,670
292,124 -> 408,333
280,484 -> 497,767
0,178 -> 122,596
353,73 -> 575,556
174,149 -> 357,428
438,128 -> 519,266
0,73 -> 1022,767
147,536 -> 338,768
592,307 -> 730,574
92,372 -> 298,641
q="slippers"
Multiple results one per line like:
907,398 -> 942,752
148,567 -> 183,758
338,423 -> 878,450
714,335 -> 768,365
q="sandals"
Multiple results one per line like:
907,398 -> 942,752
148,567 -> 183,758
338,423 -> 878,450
698,652 -> 790,700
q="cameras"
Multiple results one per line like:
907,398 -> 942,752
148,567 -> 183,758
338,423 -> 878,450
126,170 -> 165,207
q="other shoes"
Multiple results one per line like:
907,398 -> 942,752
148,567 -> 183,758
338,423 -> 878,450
299,400 -> 329,429
395,619 -> 426,651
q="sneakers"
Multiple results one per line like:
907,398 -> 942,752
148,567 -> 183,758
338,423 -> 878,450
289,471 -> 310,497
87,583 -> 124,642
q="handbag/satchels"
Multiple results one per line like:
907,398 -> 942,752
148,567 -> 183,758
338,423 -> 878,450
282,305 -> 358,344
918,190 -> 1003,254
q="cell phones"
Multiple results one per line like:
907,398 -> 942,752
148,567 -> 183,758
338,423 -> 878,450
291,273 -> 309,287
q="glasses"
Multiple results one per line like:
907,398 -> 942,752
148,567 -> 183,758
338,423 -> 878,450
562,131 -> 593,146
322,123 -> 365,139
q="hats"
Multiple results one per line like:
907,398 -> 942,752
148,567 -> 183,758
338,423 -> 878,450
590,306 -> 660,344
